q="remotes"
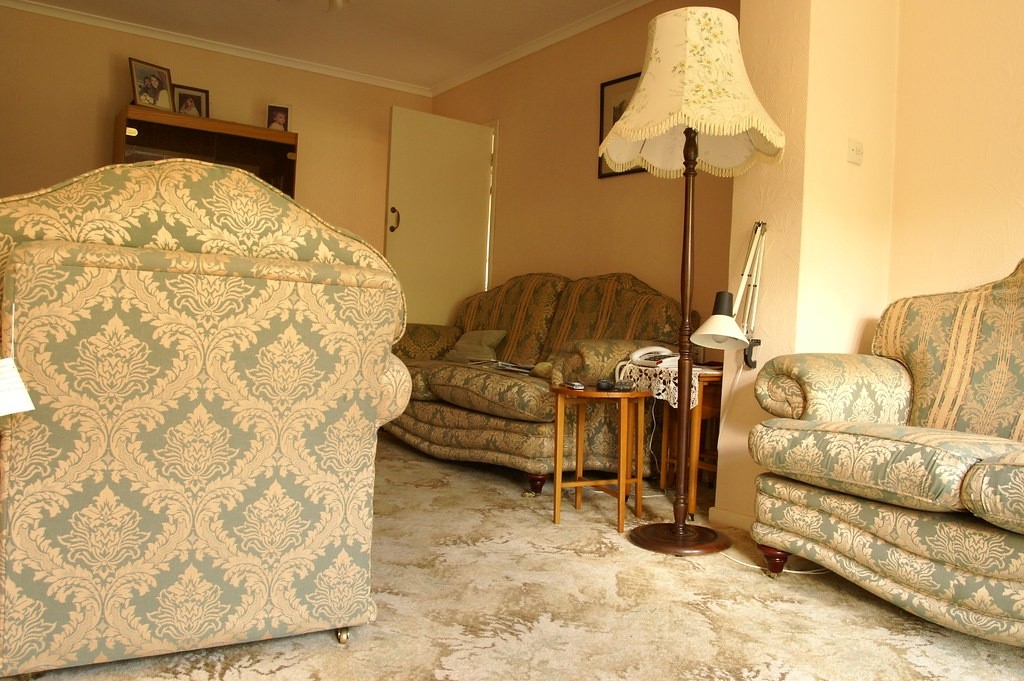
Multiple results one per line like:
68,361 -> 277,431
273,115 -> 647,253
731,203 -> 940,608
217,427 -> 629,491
598,377 -> 614,389
614,379 -> 634,390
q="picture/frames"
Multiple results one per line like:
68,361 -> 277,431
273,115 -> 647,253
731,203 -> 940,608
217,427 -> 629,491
129,58 -> 175,113
266,103 -> 290,132
597,70 -> 649,180
172,84 -> 209,119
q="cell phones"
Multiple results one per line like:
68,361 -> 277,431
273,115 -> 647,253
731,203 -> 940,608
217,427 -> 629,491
566,381 -> 584,389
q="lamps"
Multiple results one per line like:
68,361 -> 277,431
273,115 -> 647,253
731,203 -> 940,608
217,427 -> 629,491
596,7 -> 786,556
326,0 -> 352,15
688,219 -> 768,370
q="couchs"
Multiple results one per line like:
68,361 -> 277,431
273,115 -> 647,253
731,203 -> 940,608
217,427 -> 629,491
0,154 -> 414,679
381,273 -> 701,494
747,258 -> 1024,648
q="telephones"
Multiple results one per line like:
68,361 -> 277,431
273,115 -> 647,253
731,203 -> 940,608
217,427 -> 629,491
629,346 -> 681,368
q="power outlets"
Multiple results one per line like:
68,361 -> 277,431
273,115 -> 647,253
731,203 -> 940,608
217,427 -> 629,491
848,138 -> 865,166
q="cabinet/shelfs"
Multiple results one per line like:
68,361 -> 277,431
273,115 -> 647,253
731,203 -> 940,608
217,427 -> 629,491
113,104 -> 299,204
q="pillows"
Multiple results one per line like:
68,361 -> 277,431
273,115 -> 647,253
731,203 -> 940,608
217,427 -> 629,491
442,330 -> 507,362
528,347 -> 559,378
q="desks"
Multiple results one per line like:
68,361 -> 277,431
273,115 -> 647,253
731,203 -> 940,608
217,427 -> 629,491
619,364 -> 723,491
548,384 -> 654,534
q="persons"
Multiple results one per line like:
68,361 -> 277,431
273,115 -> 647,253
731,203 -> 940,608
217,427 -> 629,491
181,97 -> 199,116
268,108 -> 286,130
138,70 -> 170,108
